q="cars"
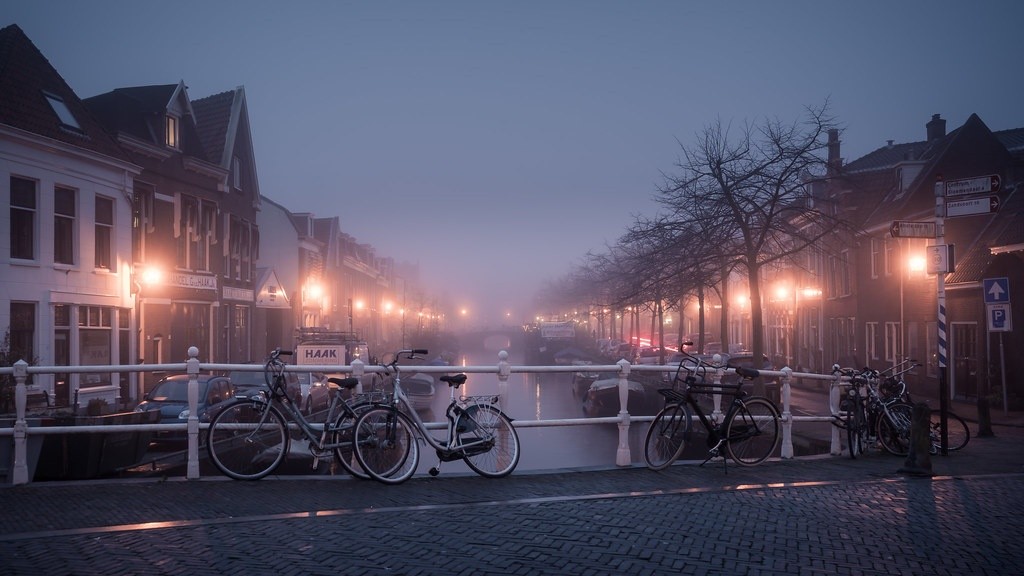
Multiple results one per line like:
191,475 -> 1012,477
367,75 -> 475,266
296,371 -> 331,416
595,331 -> 777,400
133,373 -> 238,451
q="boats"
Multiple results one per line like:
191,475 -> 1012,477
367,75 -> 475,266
581,372 -> 648,418
250,437 -> 334,476
554,347 -> 600,396
387,372 -> 435,412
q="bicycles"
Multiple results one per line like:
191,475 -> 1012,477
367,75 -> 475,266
206,347 -> 412,482
829,356 -> 971,460
352,349 -> 522,485
643,341 -> 782,475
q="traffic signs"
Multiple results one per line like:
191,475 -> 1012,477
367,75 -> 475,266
890,220 -> 936,239
946,174 -> 1002,198
946,196 -> 1000,217
927,244 -> 949,274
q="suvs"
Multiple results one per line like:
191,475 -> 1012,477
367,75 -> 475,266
220,359 -> 302,432
